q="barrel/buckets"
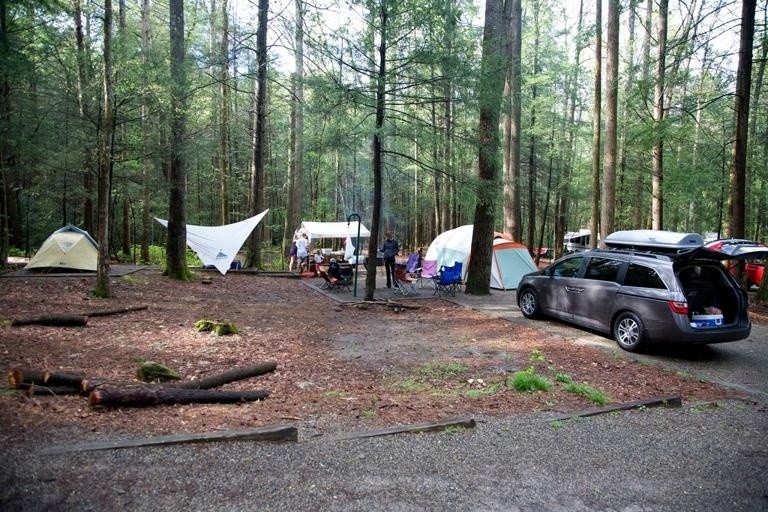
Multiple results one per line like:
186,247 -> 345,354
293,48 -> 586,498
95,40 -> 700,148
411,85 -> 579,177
745,264 -> 765,285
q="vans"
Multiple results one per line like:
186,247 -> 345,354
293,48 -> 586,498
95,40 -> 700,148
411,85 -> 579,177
516,229 -> 768,351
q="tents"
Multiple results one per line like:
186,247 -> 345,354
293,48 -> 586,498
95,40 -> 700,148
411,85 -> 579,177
423,225 -> 537,291
22,222 -> 99,271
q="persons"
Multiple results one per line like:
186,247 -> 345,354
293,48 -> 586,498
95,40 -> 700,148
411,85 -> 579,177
311,249 -> 324,271
295,234 -> 310,272
378,232 -> 400,289
289,235 -> 308,271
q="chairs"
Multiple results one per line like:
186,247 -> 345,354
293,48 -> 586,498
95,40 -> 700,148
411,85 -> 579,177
394,253 -> 463,298
319,268 -> 354,296
